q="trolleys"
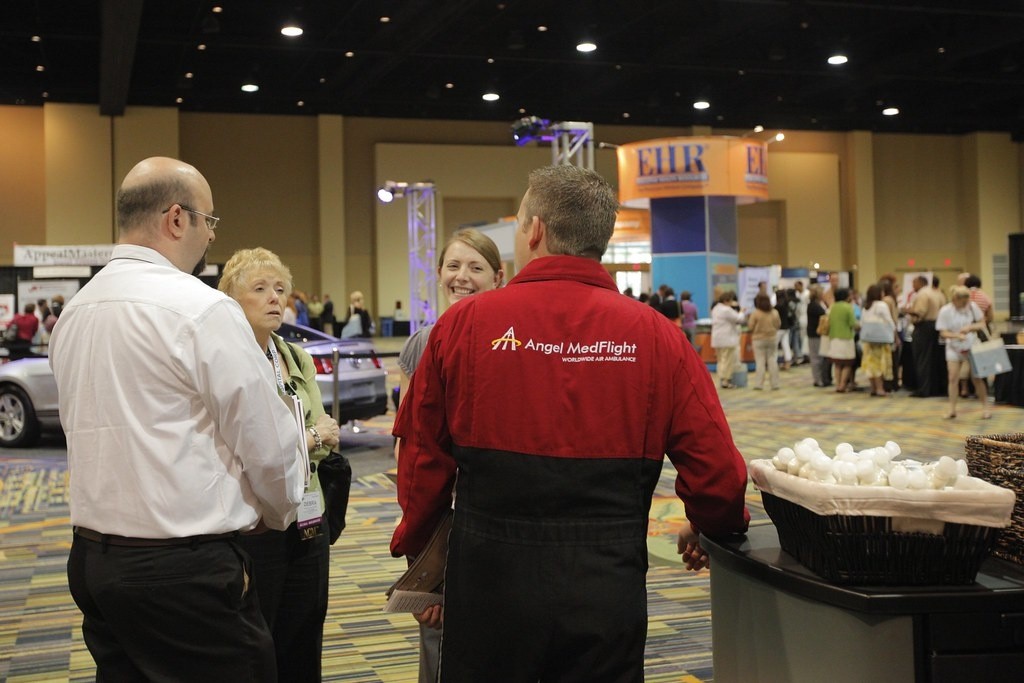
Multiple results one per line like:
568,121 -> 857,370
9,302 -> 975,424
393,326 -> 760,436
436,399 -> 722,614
698,519 -> 1024,683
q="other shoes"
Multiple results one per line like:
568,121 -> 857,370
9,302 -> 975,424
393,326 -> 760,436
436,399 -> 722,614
869,390 -> 888,397
720,381 -> 737,388
942,413 -> 957,420
835,384 -> 845,393
779,361 -> 792,371
812,381 -> 826,387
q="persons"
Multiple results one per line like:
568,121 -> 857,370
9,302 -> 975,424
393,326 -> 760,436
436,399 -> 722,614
346,291 -> 372,339
711,292 -> 747,388
958,272 -> 994,398
283,289 -> 333,335
48,156 -> 306,683
396,229 -> 504,683
806,271 -> 945,396
758,280 -> 811,370
389,166 -> 752,683
624,284 -> 697,344
748,293 -> 782,389
7,299 -> 57,359
217,246 -> 340,683
936,285 -> 993,419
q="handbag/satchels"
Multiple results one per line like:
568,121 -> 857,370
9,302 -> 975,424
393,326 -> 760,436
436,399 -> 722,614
317,450 -> 352,545
817,315 -> 830,334
6,325 -> 17,339
968,338 -> 1013,379
349,314 -> 362,335
860,322 -> 894,343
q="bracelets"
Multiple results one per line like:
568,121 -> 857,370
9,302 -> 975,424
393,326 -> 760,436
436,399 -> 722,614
308,427 -> 322,449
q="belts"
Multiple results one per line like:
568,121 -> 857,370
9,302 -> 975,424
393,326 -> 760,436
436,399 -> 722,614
74,526 -> 235,547
300,521 -> 323,542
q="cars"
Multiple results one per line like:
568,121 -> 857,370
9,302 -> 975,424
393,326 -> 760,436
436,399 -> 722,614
0,320 -> 391,449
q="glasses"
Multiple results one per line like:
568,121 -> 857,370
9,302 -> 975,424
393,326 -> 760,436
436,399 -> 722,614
163,205 -> 219,229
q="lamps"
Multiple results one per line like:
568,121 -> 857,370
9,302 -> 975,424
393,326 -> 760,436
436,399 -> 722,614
378,179 -> 407,203
509,117 -> 539,146
